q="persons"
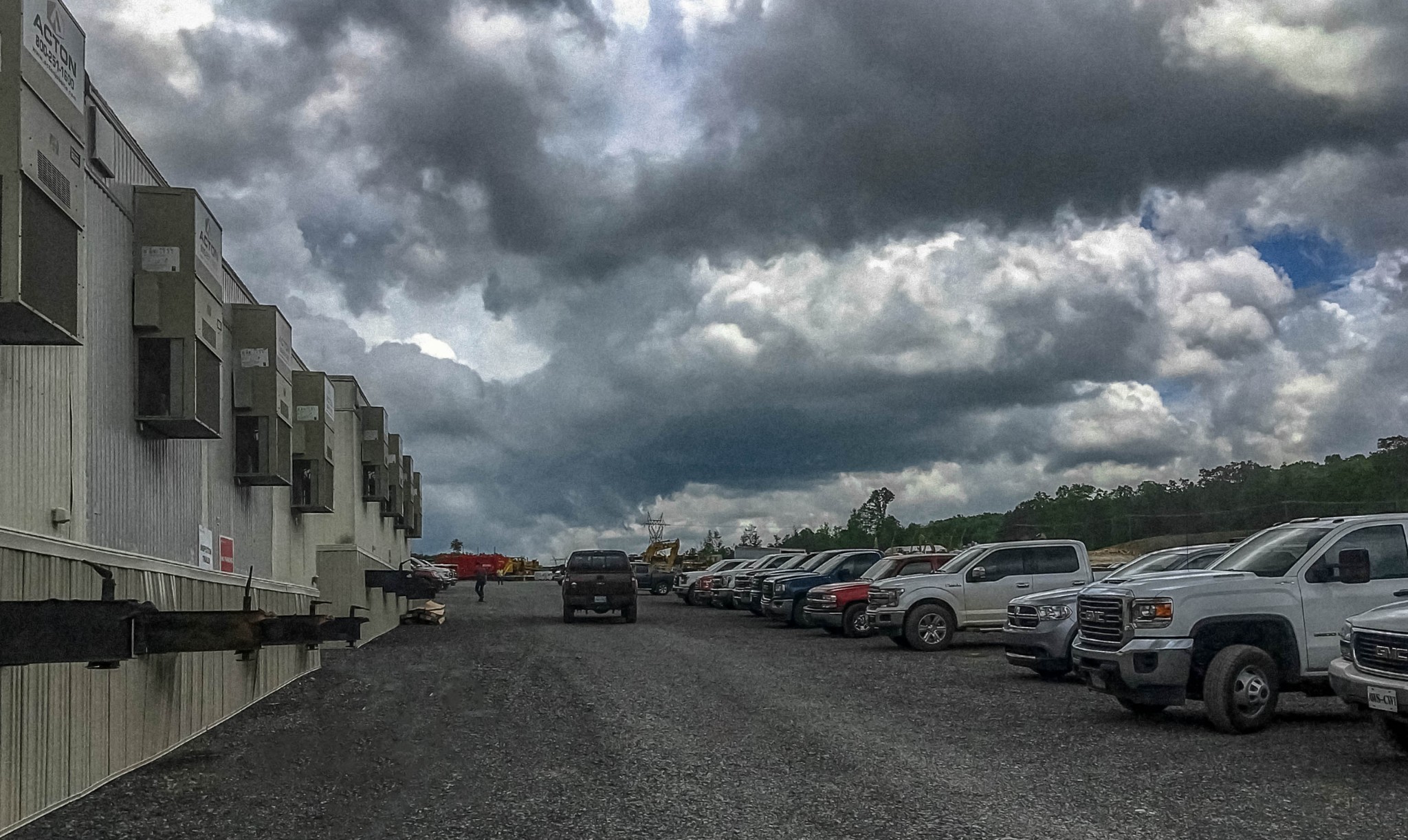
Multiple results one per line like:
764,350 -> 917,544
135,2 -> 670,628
473,562 -> 487,602
496,567 -> 504,585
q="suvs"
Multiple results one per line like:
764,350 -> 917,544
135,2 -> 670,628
410,556 -> 459,592
1074,514 -> 1408,735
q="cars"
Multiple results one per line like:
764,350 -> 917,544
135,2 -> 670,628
629,537 -> 952,629
804,554 -> 960,638
553,565 -> 565,584
1000,542 -> 1307,693
1328,600 -> 1408,741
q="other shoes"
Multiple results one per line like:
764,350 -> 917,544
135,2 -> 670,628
478,596 -> 483,602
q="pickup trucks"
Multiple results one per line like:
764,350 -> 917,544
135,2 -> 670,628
554,548 -> 639,624
864,540 -> 1128,655
